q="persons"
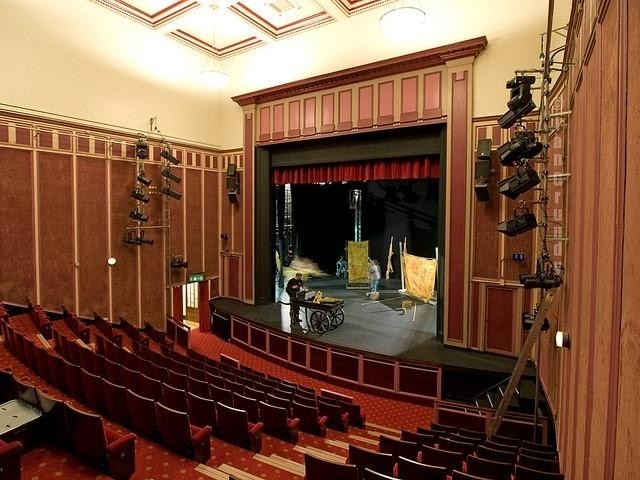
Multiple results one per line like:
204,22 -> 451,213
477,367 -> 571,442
367,257 -> 381,293
285,272 -> 309,326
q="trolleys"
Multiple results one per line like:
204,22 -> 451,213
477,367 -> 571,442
277,291 -> 347,335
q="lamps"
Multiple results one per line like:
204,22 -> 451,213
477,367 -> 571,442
226,164 -> 240,204
475,69 -> 563,332
122,132 -> 182,247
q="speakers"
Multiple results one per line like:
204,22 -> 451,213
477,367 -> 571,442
478,139 -> 493,159
225,177 -> 238,190
475,160 -> 493,179
475,184 -> 492,202
228,191 -> 239,205
226,163 -> 237,176
